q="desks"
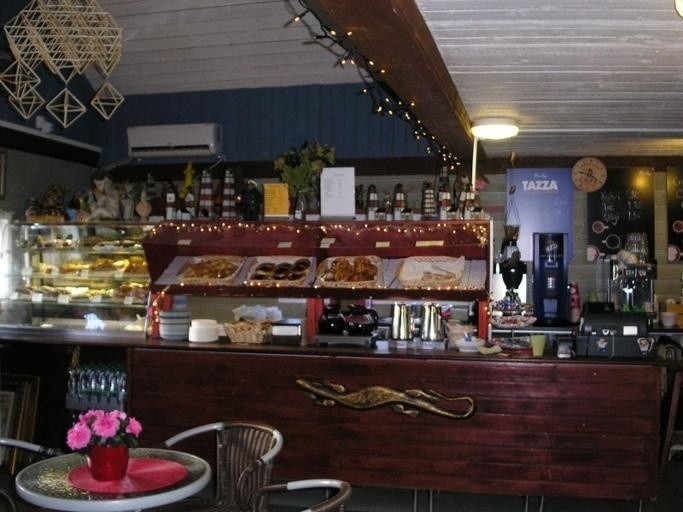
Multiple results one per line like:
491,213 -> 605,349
14,446 -> 212,512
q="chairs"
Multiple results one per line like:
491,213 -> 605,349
154,416 -> 353,511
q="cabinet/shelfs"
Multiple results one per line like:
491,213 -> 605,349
137,218 -> 494,349
1,217 -> 159,333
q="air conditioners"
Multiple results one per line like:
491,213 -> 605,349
126,123 -> 224,158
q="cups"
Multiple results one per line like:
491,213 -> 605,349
530,334 -> 546,357
568,281 -> 581,323
367,206 -> 471,221
662,312 -> 677,328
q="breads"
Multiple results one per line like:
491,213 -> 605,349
322,258 -> 377,281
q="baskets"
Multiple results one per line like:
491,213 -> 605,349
220,317 -> 272,344
395,254 -> 469,288
242,256 -> 312,287
313,252 -> 383,288
178,254 -> 245,288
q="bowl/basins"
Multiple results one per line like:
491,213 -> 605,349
158,309 -> 218,343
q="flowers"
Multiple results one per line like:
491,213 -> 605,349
67,408 -> 144,451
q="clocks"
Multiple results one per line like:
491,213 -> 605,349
572,157 -> 607,192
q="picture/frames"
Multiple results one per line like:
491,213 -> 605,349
0,377 -> 29,477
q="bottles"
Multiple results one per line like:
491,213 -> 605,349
294,190 -> 306,221
544,234 -> 558,267
499,238 -> 520,274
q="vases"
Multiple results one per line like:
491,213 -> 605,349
88,443 -> 130,482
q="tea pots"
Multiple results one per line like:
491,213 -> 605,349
319,304 -> 378,337
